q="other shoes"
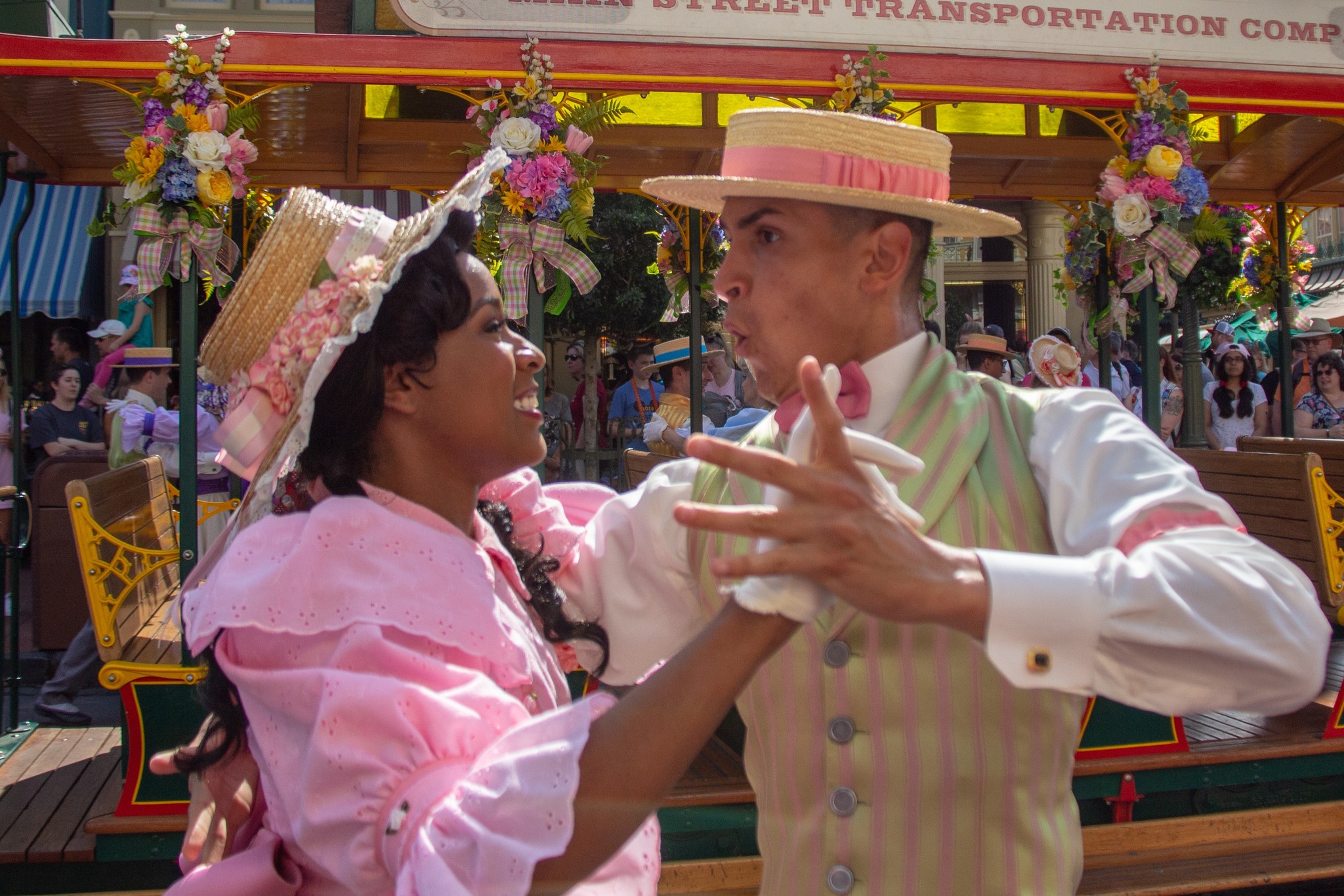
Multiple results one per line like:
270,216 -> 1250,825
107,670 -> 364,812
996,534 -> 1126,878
34,702 -> 92,724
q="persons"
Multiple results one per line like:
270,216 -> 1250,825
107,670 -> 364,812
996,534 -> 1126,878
924,318 -> 941,343
160,149 -> 930,896
0,321 -> 232,728
148,111 -> 1330,896
566,340 -> 610,482
79,263 -> 155,412
543,362 -> 575,486
643,327 -> 777,459
955,318 -> 1344,451
607,344 -> 664,494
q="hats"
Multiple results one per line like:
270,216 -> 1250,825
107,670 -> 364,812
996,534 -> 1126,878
1292,317 -> 1343,349
954,334 -> 1015,360
196,148 -> 513,538
1205,321 -> 1236,338
985,324 -> 1004,339
639,334 -> 726,376
640,107 -> 1022,238
86,319 -> 127,338
1219,343 -> 1248,361
109,347 -> 180,368
1029,334 -> 1083,389
1047,326 -> 1071,340
118,264 -> 139,286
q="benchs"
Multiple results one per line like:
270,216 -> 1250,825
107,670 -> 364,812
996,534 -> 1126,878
1165,433 -> 1344,626
60,452 -> 242,818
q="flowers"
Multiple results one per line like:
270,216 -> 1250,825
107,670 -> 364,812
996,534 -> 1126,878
1221,201 -> 1317,319
83,15 -> 270,301
643,195 -> 732,316
822,42 -> 905,121
453,35 -> 633,320
1058,66 -> 1210,315
216,251 -> 386,414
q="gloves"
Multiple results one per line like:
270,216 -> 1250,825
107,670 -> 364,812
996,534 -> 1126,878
643,412 -> 671,444
104,399 -> 129,415
718,362 -> 926,625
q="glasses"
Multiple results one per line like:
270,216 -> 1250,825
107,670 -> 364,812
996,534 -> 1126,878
1314,369 -> 1333,376
92,335 -> 115,342
565,354 -> 582,361
995,359 -> 1007,368
1302,338 -> 1328,345
1225,355 -> 1243,364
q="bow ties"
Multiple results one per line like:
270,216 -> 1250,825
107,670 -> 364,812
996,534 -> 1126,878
773,360 -> 872,434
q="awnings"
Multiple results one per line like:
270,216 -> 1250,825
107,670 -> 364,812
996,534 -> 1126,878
0,179 -> 106,319
280,188 -> 439,220
